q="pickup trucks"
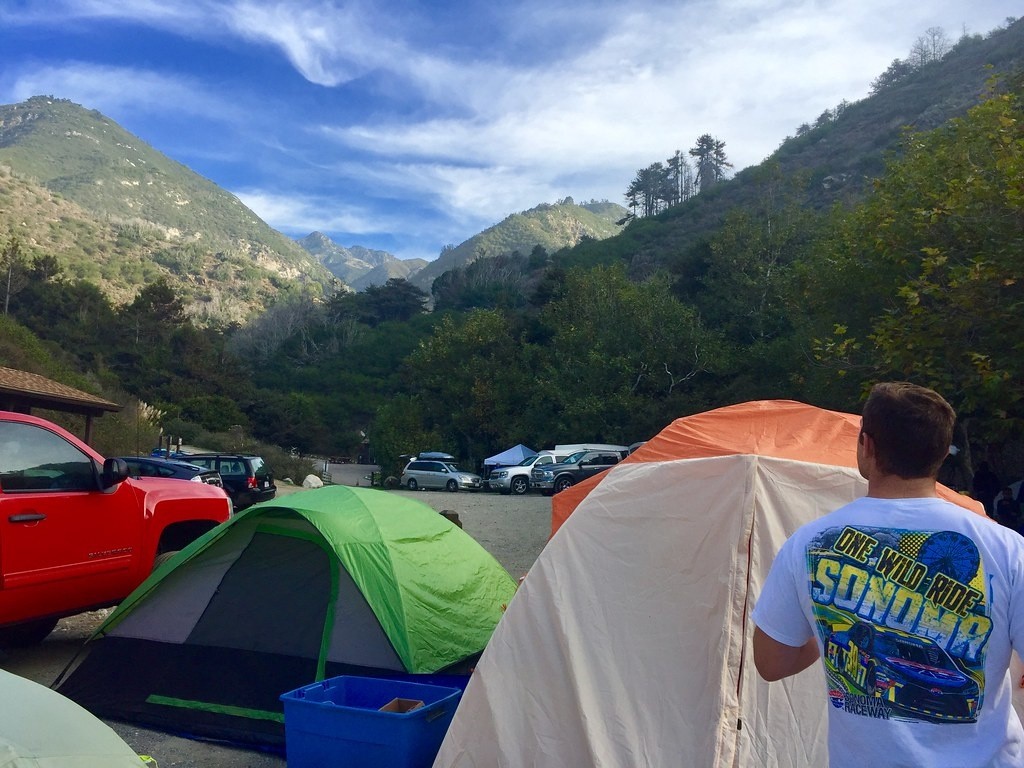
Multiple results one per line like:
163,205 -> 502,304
529,450 -> 631,496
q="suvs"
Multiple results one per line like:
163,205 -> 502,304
488,452 -> 570,495
400,453 -> 484,493
164,451 -> 277,511
1,406 -> 233,648
111,454 -> 225,490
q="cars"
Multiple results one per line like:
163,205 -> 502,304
827,616 -> 982,728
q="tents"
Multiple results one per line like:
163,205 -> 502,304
483,443 -> 537,490
1,669 -> 158,768
48,484 -> 520,764
431,400 -> 1024,767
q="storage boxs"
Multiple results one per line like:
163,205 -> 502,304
280,673 -> 462,768
377,696 -> 425,713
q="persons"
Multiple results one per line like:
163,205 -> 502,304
751,381 -> 1024,767
